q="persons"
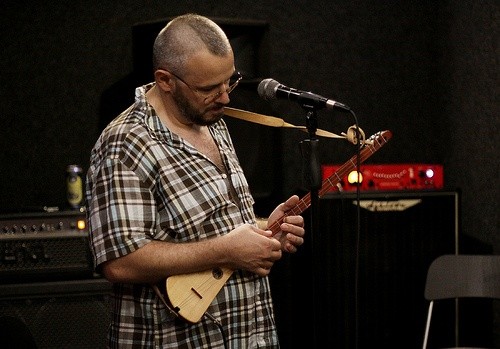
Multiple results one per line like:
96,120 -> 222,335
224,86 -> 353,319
86,14 -> 305,349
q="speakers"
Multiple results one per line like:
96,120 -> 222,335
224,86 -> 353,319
0,278 -> 116,349
130,18 -> 286,217
293,186 -> 459,349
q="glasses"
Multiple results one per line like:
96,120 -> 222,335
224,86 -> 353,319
159,67 -> 243,106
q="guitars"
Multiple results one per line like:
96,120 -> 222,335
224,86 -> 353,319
165,129 -> 393,324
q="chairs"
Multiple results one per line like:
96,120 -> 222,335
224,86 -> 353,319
423,255 -> 500,349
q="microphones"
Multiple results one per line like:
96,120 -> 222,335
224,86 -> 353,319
258,78 -> 352,114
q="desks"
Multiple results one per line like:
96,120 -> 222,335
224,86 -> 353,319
324,191 -> 458,349
